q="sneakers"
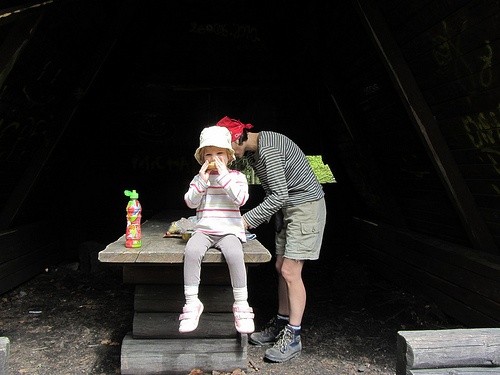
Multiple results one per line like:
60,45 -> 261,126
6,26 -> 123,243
232,302 -> 255,333
178,299 -> 204,332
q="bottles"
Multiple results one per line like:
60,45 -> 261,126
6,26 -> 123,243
124,189 -> 142,248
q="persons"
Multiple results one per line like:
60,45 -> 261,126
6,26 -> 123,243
215,115 -> 327,363
178,125 -> 256,334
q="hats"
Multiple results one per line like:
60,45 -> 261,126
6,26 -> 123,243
195,126 -> 235,167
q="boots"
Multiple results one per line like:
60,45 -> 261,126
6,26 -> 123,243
249,316 -> 289,345
264,326 -> 302,362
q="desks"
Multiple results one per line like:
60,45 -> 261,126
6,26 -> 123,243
97,207 -> 272,375
